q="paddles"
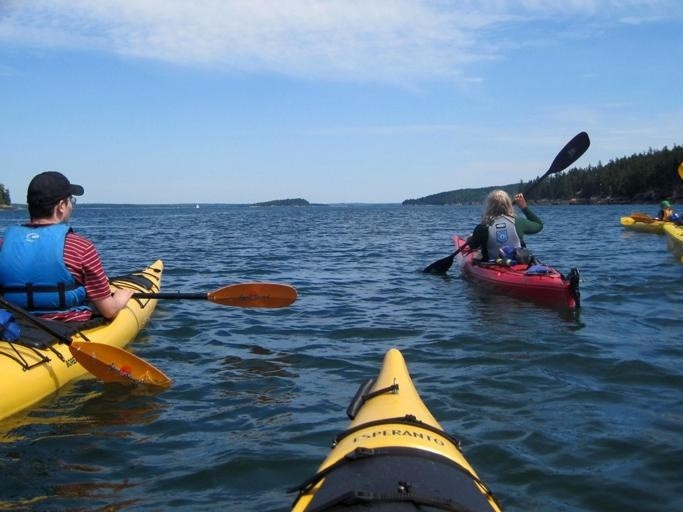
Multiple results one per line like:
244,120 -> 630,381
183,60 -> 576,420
621,216 -> 670,225
677,162 -> 683,179
1,299 -> 172,390
133,283 -> 296,307
423,132 -> 590,275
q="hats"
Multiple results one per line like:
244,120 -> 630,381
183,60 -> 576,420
27,171 -> 84,206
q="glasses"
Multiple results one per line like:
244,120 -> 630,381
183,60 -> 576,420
61,198 -> 76,204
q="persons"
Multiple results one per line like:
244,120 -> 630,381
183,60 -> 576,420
466,189 -> 544,262
659,199 -> 674,221
0,171 -> 134,324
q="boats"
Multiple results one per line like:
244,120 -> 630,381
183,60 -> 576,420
0,256 -> 166,424
290,344 -> 505,512
449,235 -> 576,311
662,218 -> 683,263
619,213 -> 671,236
